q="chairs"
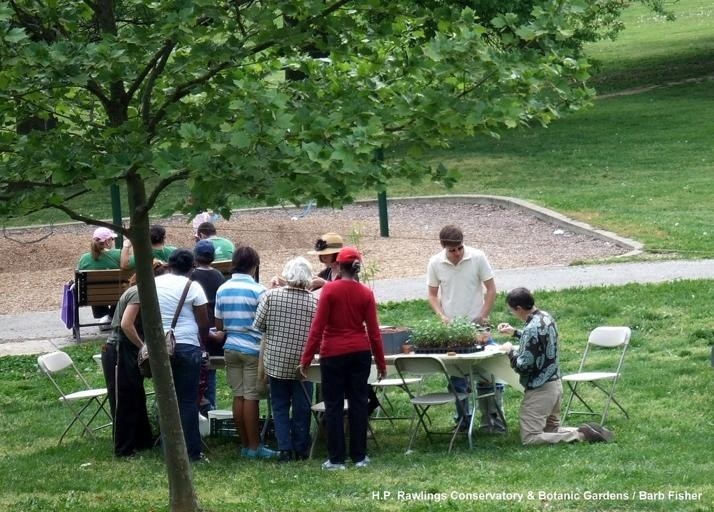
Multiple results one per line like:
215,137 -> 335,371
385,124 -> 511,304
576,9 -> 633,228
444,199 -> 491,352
38,350 -> 114,447
367,363 -> 432,436
560,327 -> 632,428
296,362 -> 382,460
394,357 -> 470,458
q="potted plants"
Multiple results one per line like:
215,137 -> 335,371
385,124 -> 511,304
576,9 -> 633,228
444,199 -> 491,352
405,313 -> 486,356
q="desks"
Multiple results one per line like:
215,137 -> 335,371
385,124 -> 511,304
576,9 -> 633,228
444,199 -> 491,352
309,340 -> 525,452
93,350 -> 272,446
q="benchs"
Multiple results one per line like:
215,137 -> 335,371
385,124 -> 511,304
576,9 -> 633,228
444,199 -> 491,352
69,261 -> 260,344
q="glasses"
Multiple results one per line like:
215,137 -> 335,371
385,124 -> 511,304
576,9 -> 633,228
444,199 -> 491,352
447,245 -> 465,252
478,327 -> 491,333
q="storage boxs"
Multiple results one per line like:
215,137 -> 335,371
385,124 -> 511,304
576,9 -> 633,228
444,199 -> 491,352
210,415 -> 292,442
369,328 -> 413,357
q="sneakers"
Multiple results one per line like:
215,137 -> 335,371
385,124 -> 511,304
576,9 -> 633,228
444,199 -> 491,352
354,456 -> 371,468
587,423 -> 613,440
578,424 -> 606,443
247,445 -> 278,459
242,448 -> 249,457
322,459 -> 346,471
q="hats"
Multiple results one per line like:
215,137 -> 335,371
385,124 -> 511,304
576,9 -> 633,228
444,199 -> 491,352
306,233 -> 346,255
193,241 -> 215,255
337,247 -> 362,263
93,227 -> 118,242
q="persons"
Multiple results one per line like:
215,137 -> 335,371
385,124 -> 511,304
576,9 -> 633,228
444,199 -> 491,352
426,225 -> 510,438
497,287 -> 616,447
153,246 -> 210,464
253,256 -> 320,462
214,246 -> 279,461
268,232 -> 360,291
75,226 -> 122,322
296,248 -> 388,473
101,257 -> 171,459
189,239 -> 225,417
118,225 -> 178,270
196,222 -> 236,261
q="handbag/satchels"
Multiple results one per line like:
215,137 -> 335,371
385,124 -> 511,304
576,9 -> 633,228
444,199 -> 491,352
138,280 -> 193,371
61,281 -> 76,330
